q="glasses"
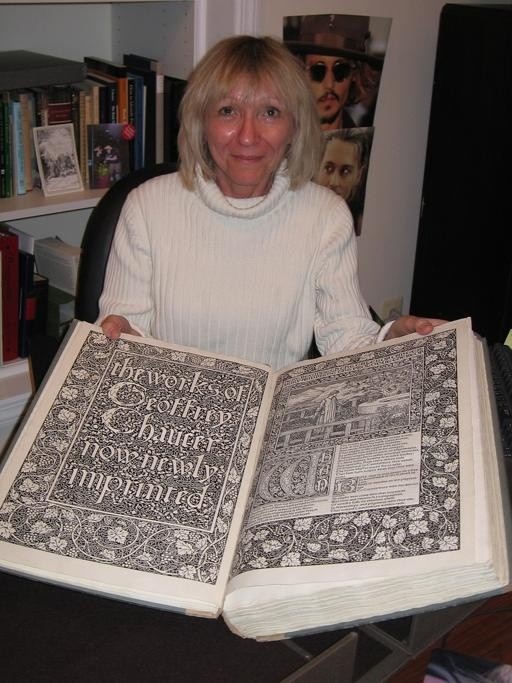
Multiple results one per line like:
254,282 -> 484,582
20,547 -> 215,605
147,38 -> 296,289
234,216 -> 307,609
305,60 -> 353,82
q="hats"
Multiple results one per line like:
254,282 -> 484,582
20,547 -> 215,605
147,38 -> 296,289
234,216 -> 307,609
283,14 -> 384,65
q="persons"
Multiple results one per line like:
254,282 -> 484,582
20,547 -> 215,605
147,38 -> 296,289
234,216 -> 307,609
101,35 -> 451,343
297,53 -> 378,235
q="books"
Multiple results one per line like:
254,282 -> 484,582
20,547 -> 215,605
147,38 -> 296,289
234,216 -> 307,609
2,222 -> 82,361
0,316 -> 512,645
0,51 -> 185,196
